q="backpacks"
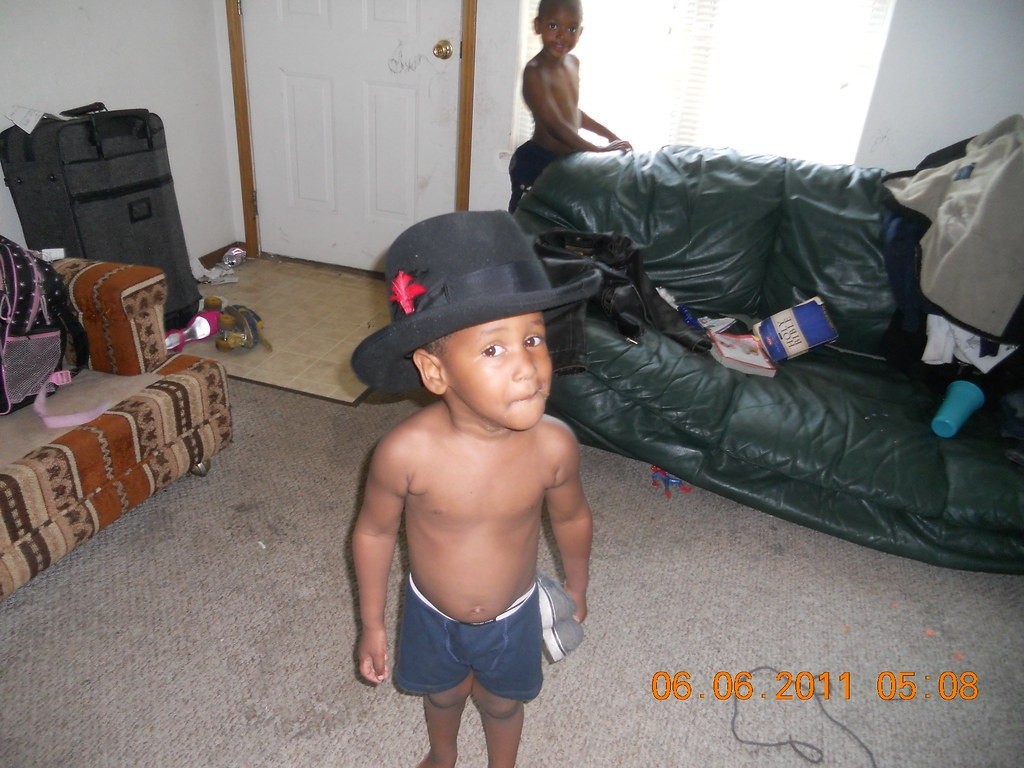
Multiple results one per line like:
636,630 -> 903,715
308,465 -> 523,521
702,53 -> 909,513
0,235 -> 88,416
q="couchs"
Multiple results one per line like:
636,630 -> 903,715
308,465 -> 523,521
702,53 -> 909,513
0,258 -> 235,601
509,142 -> 1024,577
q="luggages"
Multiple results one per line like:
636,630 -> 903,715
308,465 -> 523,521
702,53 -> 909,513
0,102 -> 202,331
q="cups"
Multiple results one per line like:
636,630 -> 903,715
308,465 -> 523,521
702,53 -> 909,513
931,381 -> 985,437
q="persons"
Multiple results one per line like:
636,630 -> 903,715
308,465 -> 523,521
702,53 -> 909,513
509,0 -> 634,215
349,211 -> 601,768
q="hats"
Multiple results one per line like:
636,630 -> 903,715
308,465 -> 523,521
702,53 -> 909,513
351,210 -> 602,393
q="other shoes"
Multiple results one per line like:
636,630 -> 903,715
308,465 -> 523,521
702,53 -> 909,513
165,311 -> 220,352
543,619 -> 584,662
537,570 -> 576,628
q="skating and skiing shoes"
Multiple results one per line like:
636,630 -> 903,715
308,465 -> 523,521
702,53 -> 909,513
204,295 -> 264,352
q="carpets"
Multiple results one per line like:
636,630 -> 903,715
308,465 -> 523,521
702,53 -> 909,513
0,372 -> 1023,768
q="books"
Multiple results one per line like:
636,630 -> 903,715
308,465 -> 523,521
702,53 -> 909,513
678,297 -> 840,377
